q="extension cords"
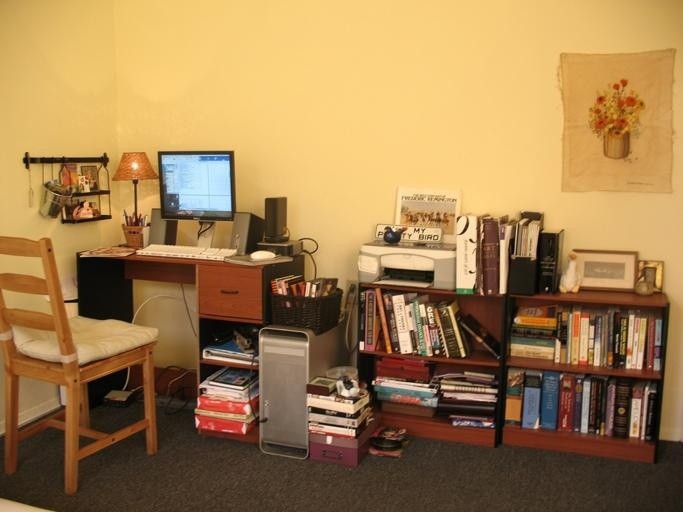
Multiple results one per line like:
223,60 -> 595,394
149,391 -> 202,414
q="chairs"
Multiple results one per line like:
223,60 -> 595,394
0,235 -> 158,495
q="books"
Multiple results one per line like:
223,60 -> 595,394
80,247 -> 135,257
510,305 -> 662,370
271,275 -> 338,308
372,378 -> 440,408
505,366 -> 657,440
432,370 -> 498,428
456,211 -> 564,295
308,389 -> 371,438
360,288 -> 501,361
198,369 -> 258,402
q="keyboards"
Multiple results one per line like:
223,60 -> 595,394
135,243 -> 237,261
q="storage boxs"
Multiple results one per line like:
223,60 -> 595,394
309,425 -> 373,467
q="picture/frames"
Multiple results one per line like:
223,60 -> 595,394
573,249 -> 638,292
638,260 -> 664,293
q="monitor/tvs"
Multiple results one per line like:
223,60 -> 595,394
158,151 -> 234,247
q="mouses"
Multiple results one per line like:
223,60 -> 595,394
250,250 -> 276,261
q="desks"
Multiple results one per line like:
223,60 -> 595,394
77,245 -> 304,406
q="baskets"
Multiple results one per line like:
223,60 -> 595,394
267,289 -> 343,335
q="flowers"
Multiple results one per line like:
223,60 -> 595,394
589,80 -> 645,138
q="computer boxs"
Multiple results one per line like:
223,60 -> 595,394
257,325 -> 353,460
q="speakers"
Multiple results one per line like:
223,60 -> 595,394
229,211 -> 265,256
150,208 -> 178,244
264,196 -> 289,242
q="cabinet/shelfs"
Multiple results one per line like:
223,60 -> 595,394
503,289 -> 669,463
357,284 -> 505,448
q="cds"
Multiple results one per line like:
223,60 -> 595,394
368,423 -> 406,459
325,366 -> 358,385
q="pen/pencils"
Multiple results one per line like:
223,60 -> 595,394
137,214 -> 143,226
143,215 -> 153,230
129,214 -> 135,225
124,211 -> 130,228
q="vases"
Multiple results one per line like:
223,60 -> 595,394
604,131 -> 630,158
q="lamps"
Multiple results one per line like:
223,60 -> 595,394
113,152 -> 158,247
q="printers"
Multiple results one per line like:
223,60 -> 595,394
357,244 -> 456,291
